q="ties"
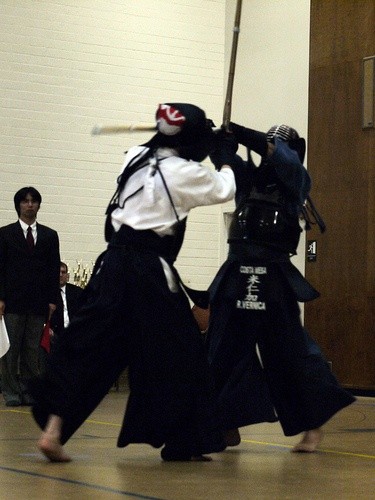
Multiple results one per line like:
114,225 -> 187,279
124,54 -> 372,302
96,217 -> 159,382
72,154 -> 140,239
26,226 -> 35,249
59,288 -> 64,328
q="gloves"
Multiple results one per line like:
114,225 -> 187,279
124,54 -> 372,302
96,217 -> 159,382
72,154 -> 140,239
209,122 -> 268,175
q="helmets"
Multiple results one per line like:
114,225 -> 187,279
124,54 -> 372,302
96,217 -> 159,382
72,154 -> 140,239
152,102 -> 216,162
264,124 -> 300,152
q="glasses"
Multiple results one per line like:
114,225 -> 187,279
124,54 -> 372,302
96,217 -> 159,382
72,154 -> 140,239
60,270 -> 67,274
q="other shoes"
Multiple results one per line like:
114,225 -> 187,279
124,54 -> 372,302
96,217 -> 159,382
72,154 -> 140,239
4,394 -> 22,407
20,394 -> 35,406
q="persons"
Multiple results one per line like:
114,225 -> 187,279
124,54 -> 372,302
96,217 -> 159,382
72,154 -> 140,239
49,261 -> 85,334
201,122 -> 356,452
30,103 -> 248,462
0,186 -> 61,407
